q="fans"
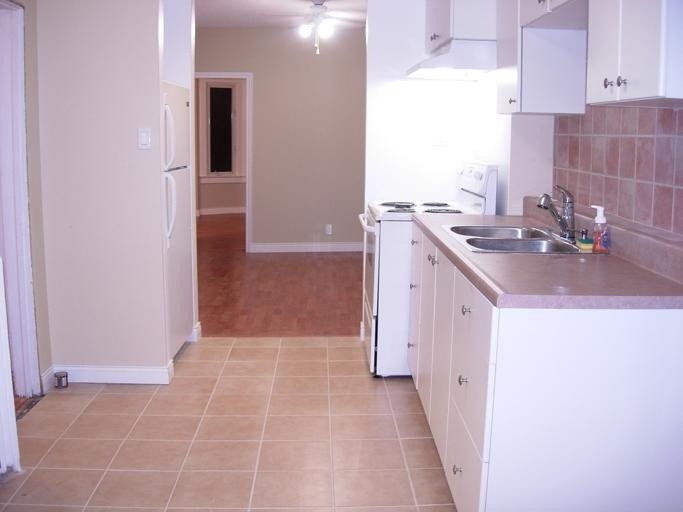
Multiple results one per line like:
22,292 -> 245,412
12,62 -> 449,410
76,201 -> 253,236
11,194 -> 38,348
264,1 -> 367,26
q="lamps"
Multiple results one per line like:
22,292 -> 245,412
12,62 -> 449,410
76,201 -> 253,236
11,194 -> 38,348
297,20 -> 335,54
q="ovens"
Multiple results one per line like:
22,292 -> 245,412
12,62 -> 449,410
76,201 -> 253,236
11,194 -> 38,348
359,210 -> 411,378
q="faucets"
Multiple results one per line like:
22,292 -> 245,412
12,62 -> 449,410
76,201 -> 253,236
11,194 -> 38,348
536,184 -> 575,238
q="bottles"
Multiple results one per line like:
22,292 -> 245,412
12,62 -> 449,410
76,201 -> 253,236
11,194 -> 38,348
53,372 -> 67,388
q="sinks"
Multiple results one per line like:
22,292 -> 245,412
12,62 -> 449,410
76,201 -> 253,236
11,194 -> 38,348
460,236 -> 584,256
441,222 -> 556,240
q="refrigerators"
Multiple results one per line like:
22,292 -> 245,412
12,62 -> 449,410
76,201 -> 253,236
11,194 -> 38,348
161,79 -> 195,362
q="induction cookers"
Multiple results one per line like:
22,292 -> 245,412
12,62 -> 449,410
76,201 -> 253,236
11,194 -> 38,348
366,198 -> 485,222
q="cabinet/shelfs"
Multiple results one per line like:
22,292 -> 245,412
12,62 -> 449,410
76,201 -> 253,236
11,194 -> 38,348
408,220 -> 424,390
520,1 -> 586,28
587,0 -> 683,107
495,0 -> 586,116
438,268 -> 681,512
425,0 -> 494,52
414,234 -> 455,468
